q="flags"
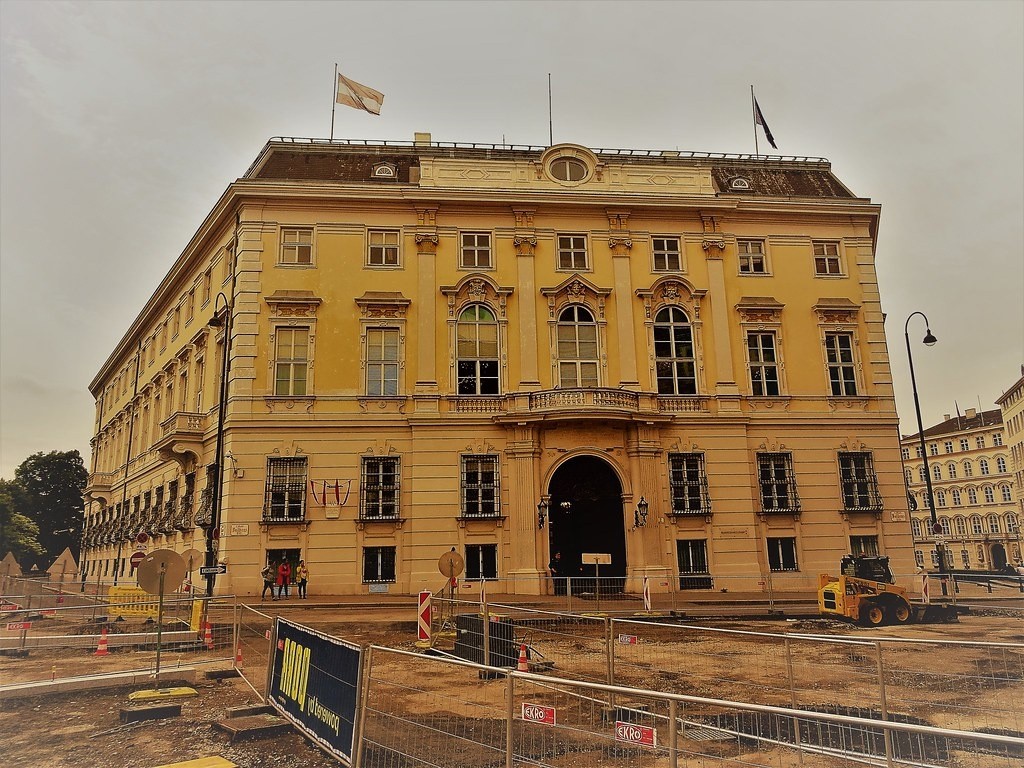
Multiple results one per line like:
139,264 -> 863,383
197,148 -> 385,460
753,95 -> 777,150
335,74 -> 385,115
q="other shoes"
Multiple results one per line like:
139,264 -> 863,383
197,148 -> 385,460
299,597 -> 302,599
303,597 -> 306,599
285,597 -> 289,600
262,598 -> 266,601
278,597 -> 281,600
272,597 -> 277,601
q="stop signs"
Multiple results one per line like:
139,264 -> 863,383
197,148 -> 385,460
130,551 -> 146,567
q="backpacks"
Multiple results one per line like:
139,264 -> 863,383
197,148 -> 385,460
263,566 -> 268,575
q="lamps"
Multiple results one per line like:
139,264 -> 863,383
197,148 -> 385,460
635,496 -> 648,527
537,496 -> 547,529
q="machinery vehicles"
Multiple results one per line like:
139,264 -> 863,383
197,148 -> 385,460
816,553 -> 969,626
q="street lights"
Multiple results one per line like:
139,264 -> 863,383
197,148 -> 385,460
905,311 -> 948,597
205,291 -> 230,601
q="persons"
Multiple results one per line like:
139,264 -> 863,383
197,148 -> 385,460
296,560 -> 309,599
278,558 -> 291,600
549,550 -> 562,596
261,560 -> 277,601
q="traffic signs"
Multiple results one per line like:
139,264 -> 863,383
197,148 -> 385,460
200,566 -> 227,575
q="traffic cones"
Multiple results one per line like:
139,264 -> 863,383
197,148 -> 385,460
236,643 -> 243,661
95,629 -> 109,656
204,620 -> 212,644
514,644 -> 529,672
